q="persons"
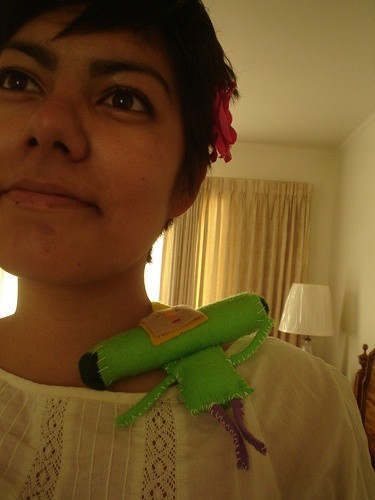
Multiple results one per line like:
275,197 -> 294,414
1,2 -> 375,495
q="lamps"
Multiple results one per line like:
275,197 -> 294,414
278,282 -> 336,356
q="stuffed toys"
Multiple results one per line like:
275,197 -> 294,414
79,291 -> 274,472
